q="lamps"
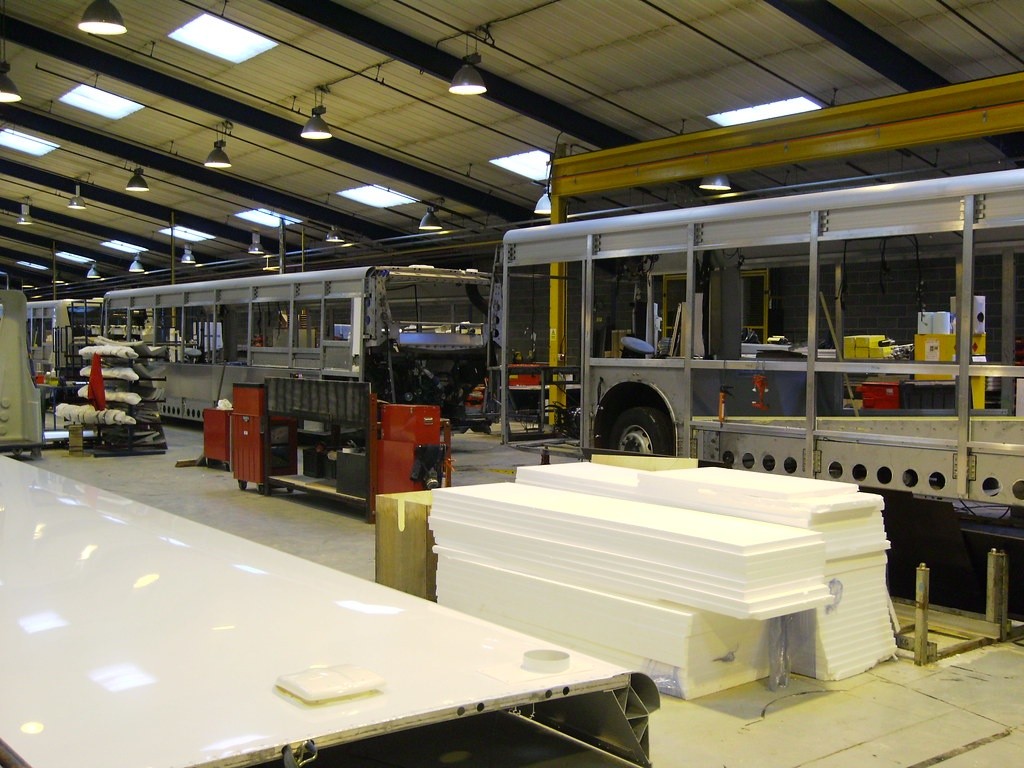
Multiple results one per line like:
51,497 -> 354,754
87,264 -> 102,279
204,122 -> 232,169
52,271 -> 65,284
67,178 -> 88,209
534,187 -> 552,215
125,168 -> 150,191
325,225 -> 346,242
699,174 -> 731,191
180,244 -> 196,264
129,252 -> 145,273
16,197 -> 34,224
78,0 -> 127,37
300,86 -> 333,140
449,29 -> 488,96
0,0 -> 23,103
248,233 -> 266,255
419,206 -> 443,230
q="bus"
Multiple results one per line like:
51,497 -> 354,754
0,297 -> 105,410
100,265 -> 503,449
501,168 -> 1024,507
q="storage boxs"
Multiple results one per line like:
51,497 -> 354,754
861,380 -> 955,410
843,334 -> 891,361
